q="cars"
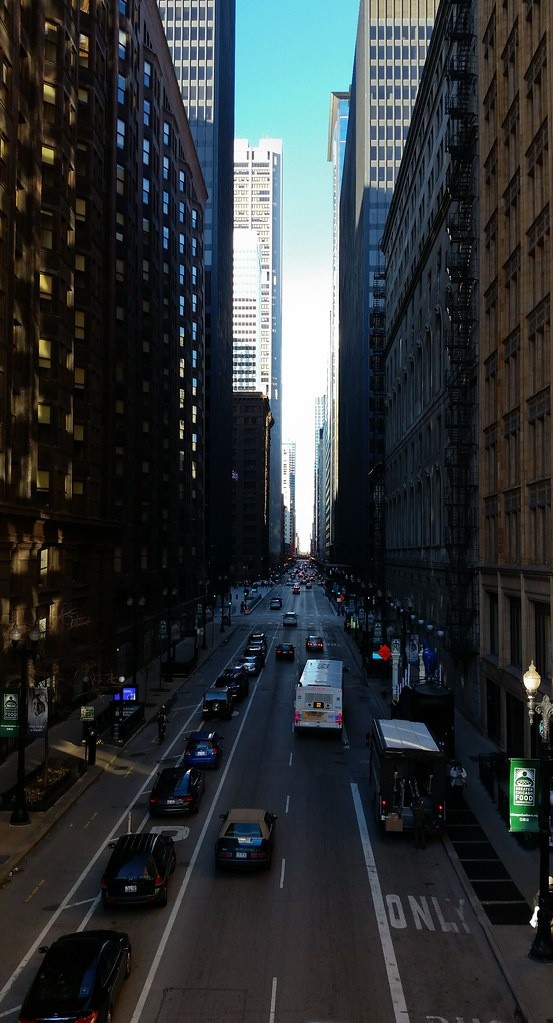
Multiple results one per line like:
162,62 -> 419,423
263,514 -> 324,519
149,767 -> 204,818
286,564 -> 316,594
275,642 -> 296,661
19,930 -> 131,1023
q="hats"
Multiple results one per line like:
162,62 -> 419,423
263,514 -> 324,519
548,876 -> 553,885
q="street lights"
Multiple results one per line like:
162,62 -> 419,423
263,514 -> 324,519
522,659 -> 553,963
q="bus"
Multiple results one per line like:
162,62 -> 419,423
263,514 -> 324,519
294,659 -> 350,731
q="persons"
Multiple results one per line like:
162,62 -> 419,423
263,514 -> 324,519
449,760 -> 470,801
157,711 -> 167,737
410,799 -> 427,850
28,690 -> 48,732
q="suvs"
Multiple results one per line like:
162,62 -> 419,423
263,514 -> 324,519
239,633 -> 268,675
202,663 -> 249,721
215,809 -> 278,872
184,732 -> 224,770
101,833 -> 176,910
282,612 -> 298,627
269,597 -> 283,609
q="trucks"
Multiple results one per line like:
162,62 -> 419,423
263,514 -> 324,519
368,718 -> 446,834
